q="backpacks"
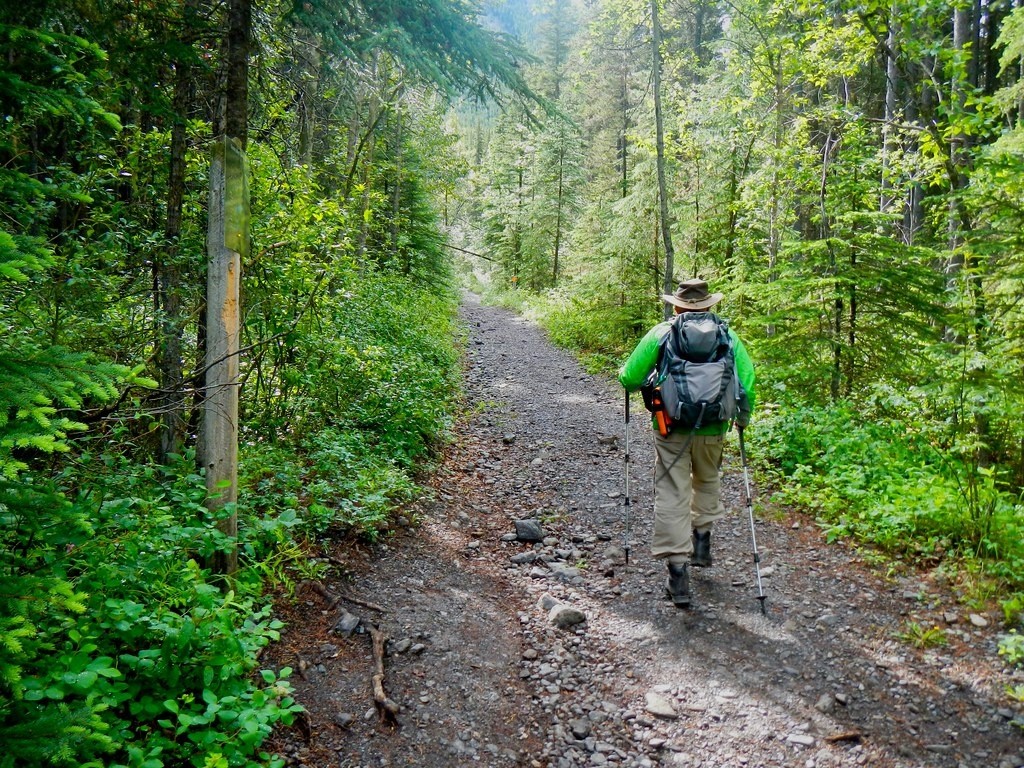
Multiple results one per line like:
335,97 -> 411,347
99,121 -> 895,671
651,311 -> 745,430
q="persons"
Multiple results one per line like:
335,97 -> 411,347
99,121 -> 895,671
617,280 -> 755,605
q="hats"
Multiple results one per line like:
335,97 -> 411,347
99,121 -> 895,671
662,277 -> 723,310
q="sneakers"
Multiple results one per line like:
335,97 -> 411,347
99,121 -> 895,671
663,561 -> 692,605
691,528 -> 714,568
